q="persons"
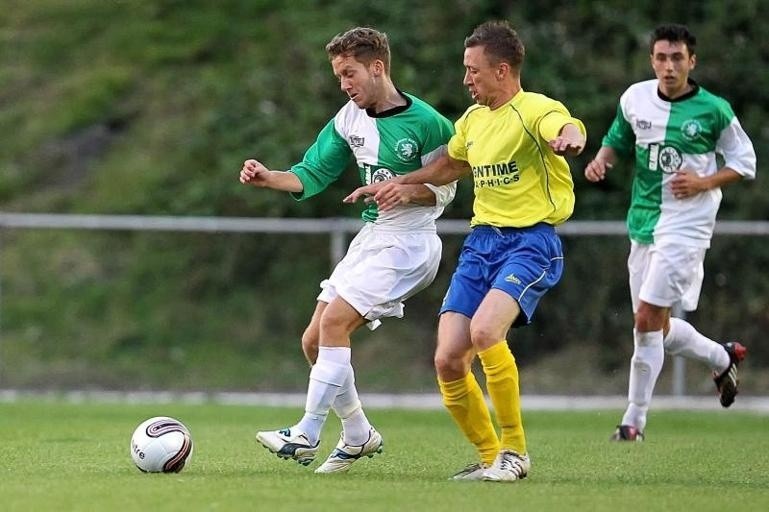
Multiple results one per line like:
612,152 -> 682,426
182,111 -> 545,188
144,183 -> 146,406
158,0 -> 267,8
240,27 -> 456,474
342,18 -> 588,482
584,25 -> 758,440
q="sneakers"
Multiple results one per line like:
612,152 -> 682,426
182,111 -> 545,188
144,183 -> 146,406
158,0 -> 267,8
256,425 -> 322,467
313,422 -> 385,474
611,422 -> 645,444
447,450 -> 531,482
712,340 -> 746,408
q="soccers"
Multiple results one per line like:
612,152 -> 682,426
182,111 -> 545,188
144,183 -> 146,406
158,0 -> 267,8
131,417 -> 192,474
